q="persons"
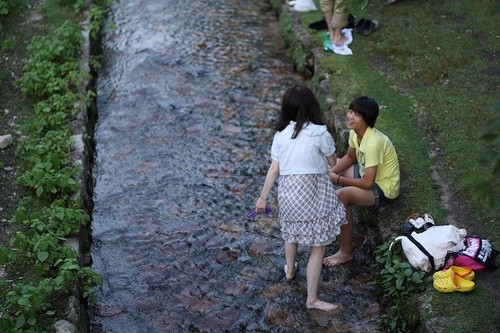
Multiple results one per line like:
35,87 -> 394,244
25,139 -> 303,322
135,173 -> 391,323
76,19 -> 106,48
319,0 -> 349,45
321,95 -> 401,267
255,84 -> 349,312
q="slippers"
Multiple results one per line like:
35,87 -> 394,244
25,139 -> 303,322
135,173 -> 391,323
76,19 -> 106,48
401,211 -> 436,232
433,265 -> 475,293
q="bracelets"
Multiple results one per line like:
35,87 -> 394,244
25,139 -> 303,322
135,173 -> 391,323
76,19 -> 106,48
260,196 -> 266,200
337,175 -> 342,184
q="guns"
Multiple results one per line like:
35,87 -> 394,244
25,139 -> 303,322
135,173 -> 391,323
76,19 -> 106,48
247,207 -> 272,218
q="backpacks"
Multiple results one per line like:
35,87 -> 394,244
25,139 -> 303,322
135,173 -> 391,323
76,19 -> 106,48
389,212 -> 492,271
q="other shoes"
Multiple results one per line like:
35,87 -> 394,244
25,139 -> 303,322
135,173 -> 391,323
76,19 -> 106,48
356,16 -> 378,36
